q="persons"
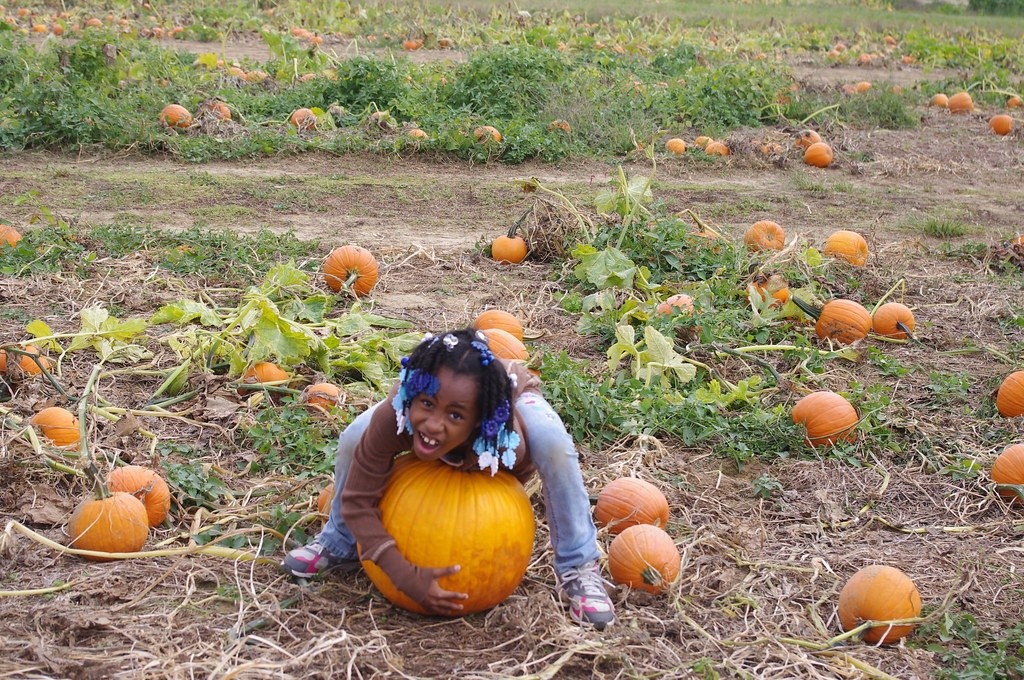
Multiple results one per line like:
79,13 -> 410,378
279,327 -> 618,632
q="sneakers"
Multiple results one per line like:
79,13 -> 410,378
555,557 -> 616,630
284,538 -> 361,578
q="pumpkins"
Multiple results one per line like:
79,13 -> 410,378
0,0 -> 1024,644
